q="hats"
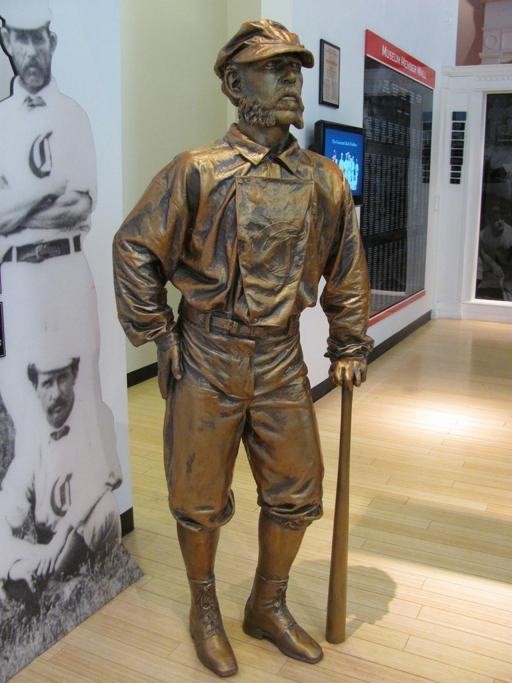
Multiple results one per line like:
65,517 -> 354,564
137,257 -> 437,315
25,345 -> 78,380
0,1 -> 53,31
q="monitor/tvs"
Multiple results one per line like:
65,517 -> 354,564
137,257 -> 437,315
313,119 -> 366,206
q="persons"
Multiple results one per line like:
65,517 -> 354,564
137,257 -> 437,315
472,203 -> 511,301
328,147 -> 360,192
1,0 -> 103,440
1,330 -> 124,622
113,18 -> 373,677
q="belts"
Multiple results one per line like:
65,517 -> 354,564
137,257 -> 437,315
2,235 -> 81,264
